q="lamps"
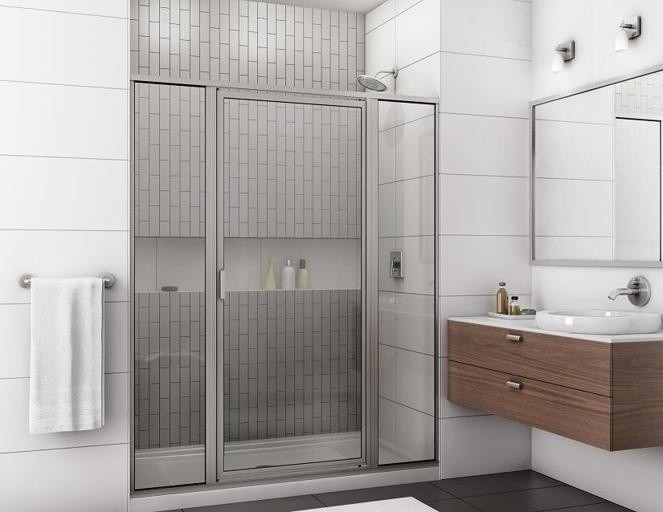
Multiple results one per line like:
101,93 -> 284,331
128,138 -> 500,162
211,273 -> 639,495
611,13 -> 642,53
546,38 -> 576,73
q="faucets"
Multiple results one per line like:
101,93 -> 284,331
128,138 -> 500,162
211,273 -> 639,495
608,280 -> 641,301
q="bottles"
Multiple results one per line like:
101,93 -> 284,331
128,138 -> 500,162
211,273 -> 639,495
521,309 -> 537,315
282,258 -> 294,290
298,257 -> 309,289
263,260 -> 276,291
508,294 -> 519,315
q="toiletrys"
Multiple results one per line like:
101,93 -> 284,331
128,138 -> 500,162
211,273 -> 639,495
297,258 -> 308,290
508,296 -> 521,315
282,259 -> 296,289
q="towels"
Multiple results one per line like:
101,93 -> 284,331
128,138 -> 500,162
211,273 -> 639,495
26,273 -> 108,439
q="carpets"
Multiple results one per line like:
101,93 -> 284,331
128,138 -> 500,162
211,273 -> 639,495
279,494 -> 441,512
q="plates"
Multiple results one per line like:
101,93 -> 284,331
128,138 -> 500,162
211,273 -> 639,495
487,311 -> 537,320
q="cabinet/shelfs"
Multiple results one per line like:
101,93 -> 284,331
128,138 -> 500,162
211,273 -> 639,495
446,316 -> 661,454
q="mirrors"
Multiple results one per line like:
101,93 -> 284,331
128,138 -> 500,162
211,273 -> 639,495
526,62 -> 662,270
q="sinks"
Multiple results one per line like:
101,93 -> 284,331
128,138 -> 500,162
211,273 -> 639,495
535,305 -> 661,336
